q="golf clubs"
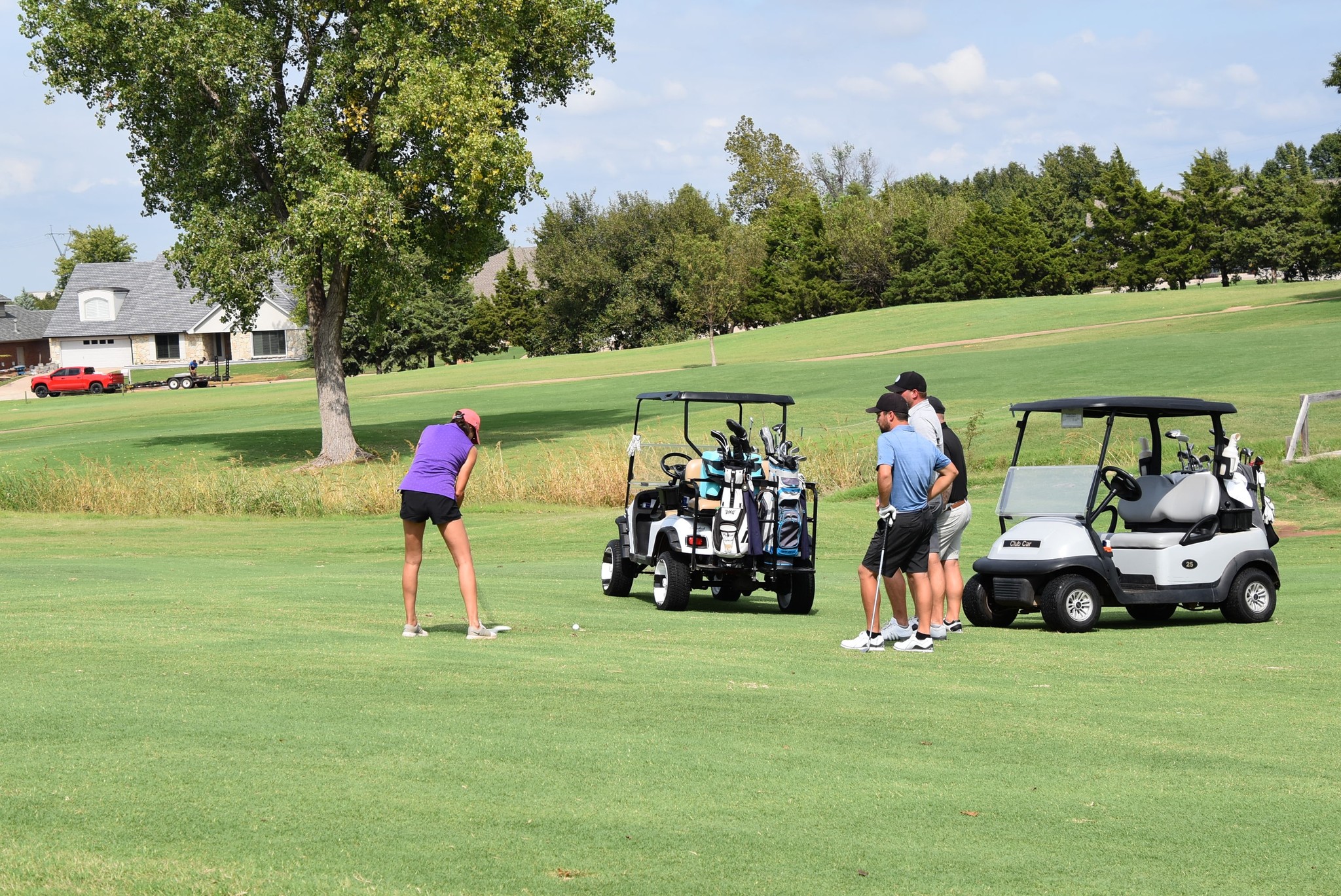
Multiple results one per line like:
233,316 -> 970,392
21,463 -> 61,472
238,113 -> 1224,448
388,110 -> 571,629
861,512 -> 890,654
744,416 -> 807,466
476,585 -> 510,632
1164,428 -> 1265,472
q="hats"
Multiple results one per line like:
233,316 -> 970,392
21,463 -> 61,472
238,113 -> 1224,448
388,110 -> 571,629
926,395 -> 946,414
452,408 -> 480,445
885,371 -> 926,392
866,393 -> 908,414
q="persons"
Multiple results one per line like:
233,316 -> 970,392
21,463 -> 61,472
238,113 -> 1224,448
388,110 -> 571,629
397,408 -> 497,640
189,360 -> 198,377
1197,281 -> 1201,289
840,372 -> 971,652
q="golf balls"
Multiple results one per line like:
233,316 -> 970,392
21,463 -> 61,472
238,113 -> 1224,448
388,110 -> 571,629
573,624 -> 579,630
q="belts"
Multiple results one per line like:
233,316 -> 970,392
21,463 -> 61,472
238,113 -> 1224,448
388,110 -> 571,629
944,497 -> 967,513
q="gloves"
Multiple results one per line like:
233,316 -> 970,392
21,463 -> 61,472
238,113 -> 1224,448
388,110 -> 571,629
879,502 -> 896,527
940,503 -> 952,514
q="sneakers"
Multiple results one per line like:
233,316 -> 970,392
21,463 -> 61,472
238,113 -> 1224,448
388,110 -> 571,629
840,630 -> 886,651
908,616 -> 921,634
893,630 -> 935,653
943,618 -> 963,633
928,623 -> 947,641
402,621 -> 429,637
880,616 -> 913,640
466,626 -> 497,640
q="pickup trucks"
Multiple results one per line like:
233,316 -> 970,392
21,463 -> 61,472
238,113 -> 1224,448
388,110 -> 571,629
31,367 -> 125,399
